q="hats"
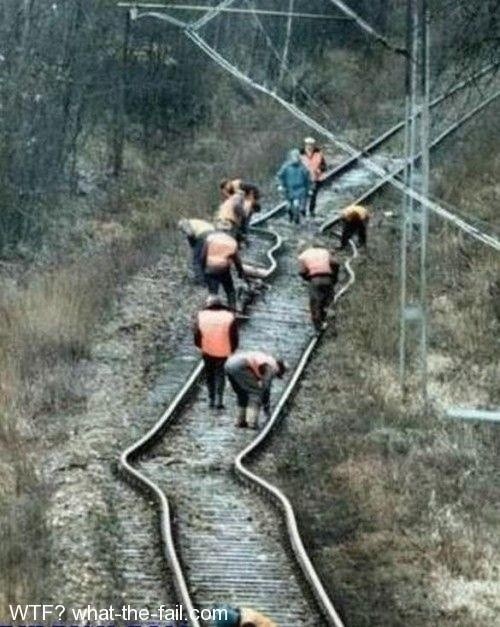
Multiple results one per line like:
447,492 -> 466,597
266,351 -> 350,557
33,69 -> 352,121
217,220 -> 233,230
304,137 -> 316,143
206,295 -> 229,308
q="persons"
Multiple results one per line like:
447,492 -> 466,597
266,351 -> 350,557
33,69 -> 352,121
209,603 -> 280,627
193,224 -> 254,315
190,293 -> 242,413
272,145 -> 316,226
294,244 -> 343,334
173,214 -> 219,287
332,202 -> 374,251
220,349 -> 294,433
298,134 -> 331,218
209,174 -> 263,250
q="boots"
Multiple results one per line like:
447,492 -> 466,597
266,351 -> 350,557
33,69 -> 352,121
233,406 -> 260,429
